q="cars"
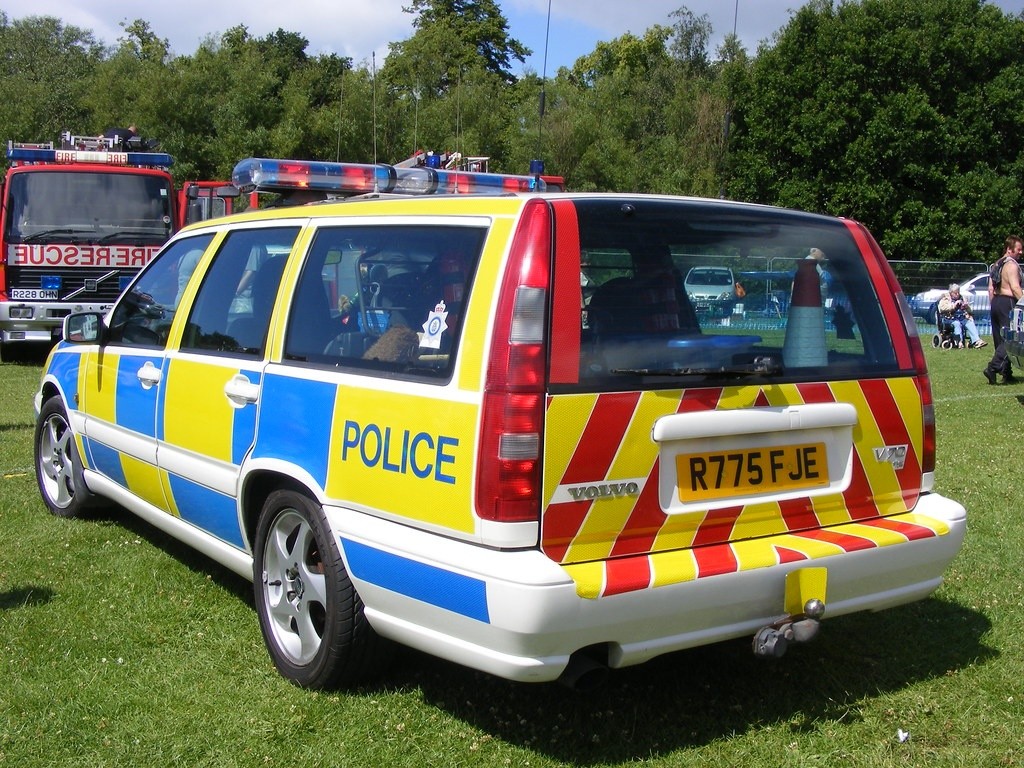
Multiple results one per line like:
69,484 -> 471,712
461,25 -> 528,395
912,272 -> 992,324
684,265 -> 736,317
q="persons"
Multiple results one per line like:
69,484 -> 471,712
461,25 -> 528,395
97,126 -> 138,148
580,271 -> 595,321
224,245 -> 268,336
938,284 -> 988,349
174,249 -> 205,311
790,248 -> 833,304
983,235 -> 1023,386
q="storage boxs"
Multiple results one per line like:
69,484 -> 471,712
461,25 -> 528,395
604,333 -> 763,374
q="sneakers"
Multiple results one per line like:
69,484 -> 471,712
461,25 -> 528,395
955,341 -> 964,348
975,339 -> 988,348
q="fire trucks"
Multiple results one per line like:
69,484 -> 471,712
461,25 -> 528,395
0,131 -> 235,360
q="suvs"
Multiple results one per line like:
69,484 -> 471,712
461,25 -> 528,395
32,158 -> 968,691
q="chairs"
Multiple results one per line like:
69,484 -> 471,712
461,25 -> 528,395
587,275 -> 644,334
229,255 -> 350,358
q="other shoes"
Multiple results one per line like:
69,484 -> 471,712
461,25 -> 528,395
1003,375 -> 1018,383
983,368 -> 996,384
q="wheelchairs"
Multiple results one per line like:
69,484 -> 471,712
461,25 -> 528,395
932,301 -> 974,350
